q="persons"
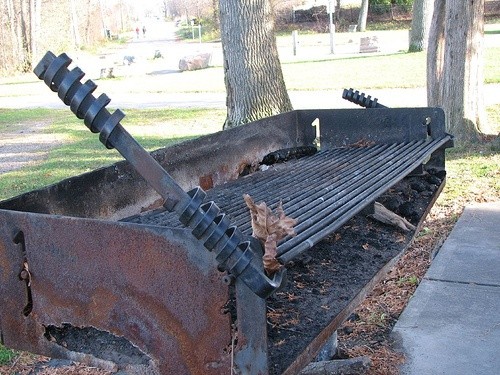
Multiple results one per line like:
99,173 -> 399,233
136,27 -> 139,37
142,26 -> 146,36
